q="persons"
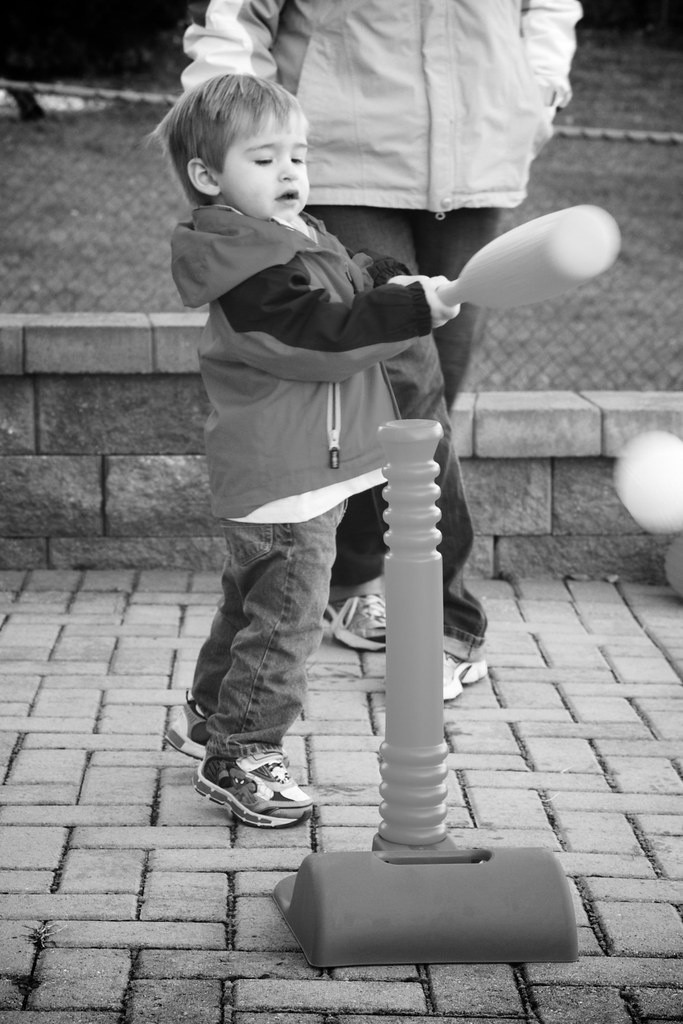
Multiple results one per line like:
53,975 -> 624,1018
176,0 -> 584,701
148,74 -> 463,827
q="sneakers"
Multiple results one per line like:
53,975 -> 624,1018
191,744 -> 313,830
442,644 -> 489,706
168,697 -> 218,757
321,592 -> 390,651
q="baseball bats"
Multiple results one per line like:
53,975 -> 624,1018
434,203 -> 620,310
